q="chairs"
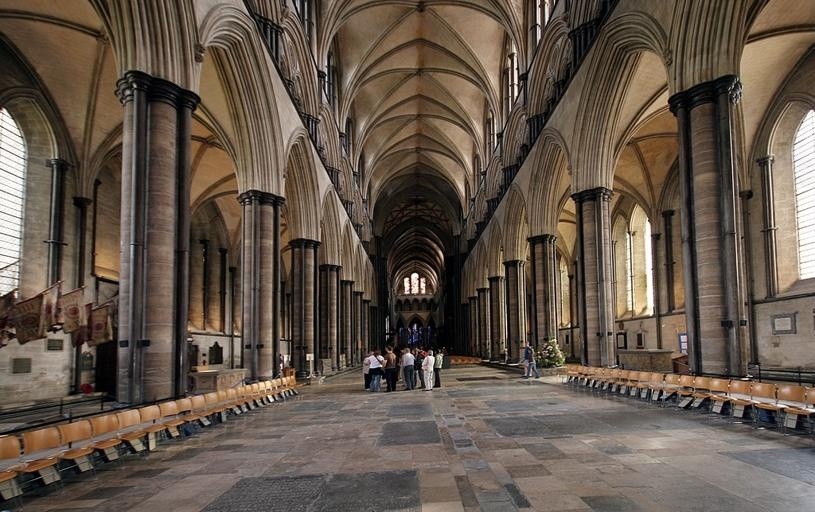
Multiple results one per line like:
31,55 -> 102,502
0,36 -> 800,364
558,364 -> 815,435
1,374 -> 307,512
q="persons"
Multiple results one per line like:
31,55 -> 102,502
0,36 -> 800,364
524,346 -> 540,379
362,342 -> 450,393
521,341 -> 532,377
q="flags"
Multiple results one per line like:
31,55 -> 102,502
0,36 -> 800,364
0,281 -> 115,348
